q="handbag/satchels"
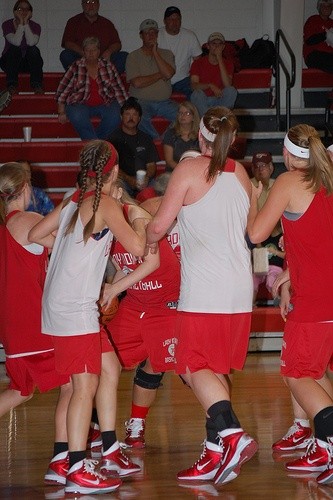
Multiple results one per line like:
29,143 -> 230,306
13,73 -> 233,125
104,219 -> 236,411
248,34 -> 276,69
201,38 -> 250,72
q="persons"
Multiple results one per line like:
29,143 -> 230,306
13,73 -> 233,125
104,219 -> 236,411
156,5 -> 202,101
15,160 -> 54,216
249,152 -> 284,251
301,0 -> 332,74
246,126 -> 332,484
107,97 -> 157,196
188,32 -> 236,118
0,0 -> 45,94
59,0 -> 128,76
124,19 -> 180,140
0,163 -> 73,486
251,222 -> 286,306
85,183 -> 190,448
272,373 -> 312,450
27,141 -> 149,494
156,102 -> 201,194
54,38 -> 129,141
143,107 -> 258,486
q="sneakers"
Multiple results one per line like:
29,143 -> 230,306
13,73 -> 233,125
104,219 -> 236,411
316,437 -> 333,487
176,438 -> 224,480
272,418 -> 313,452
99,440 -> 141,479
88,426 -> 132,459
64,458 -> 122,494
283,438 -> 332,472
125,418 -> 146,448
213,428 -> 257,486
44,449 -> 98,485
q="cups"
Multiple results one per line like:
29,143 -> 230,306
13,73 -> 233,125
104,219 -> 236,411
22,124 -> 33,142
136,170 -> 145,188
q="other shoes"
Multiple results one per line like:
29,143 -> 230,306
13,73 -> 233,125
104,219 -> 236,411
34,87 -> 44,95
8,86 -> 18,96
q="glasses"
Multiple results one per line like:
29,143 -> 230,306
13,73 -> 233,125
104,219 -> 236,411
177,111 -> 194,116
81,0 -> 99,5
14,7 -> 30,12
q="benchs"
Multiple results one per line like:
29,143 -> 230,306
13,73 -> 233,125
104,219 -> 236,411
0,68 -> 333,363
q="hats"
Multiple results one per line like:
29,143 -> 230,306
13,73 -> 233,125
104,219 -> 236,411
140,19 -> 159,33
208,32 -> 225,44
165,6 -> 181,18
253,151 -> 272,163
179,150 -> 201,163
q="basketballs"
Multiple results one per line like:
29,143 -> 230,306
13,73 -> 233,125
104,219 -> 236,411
96,294 -> 120,324
